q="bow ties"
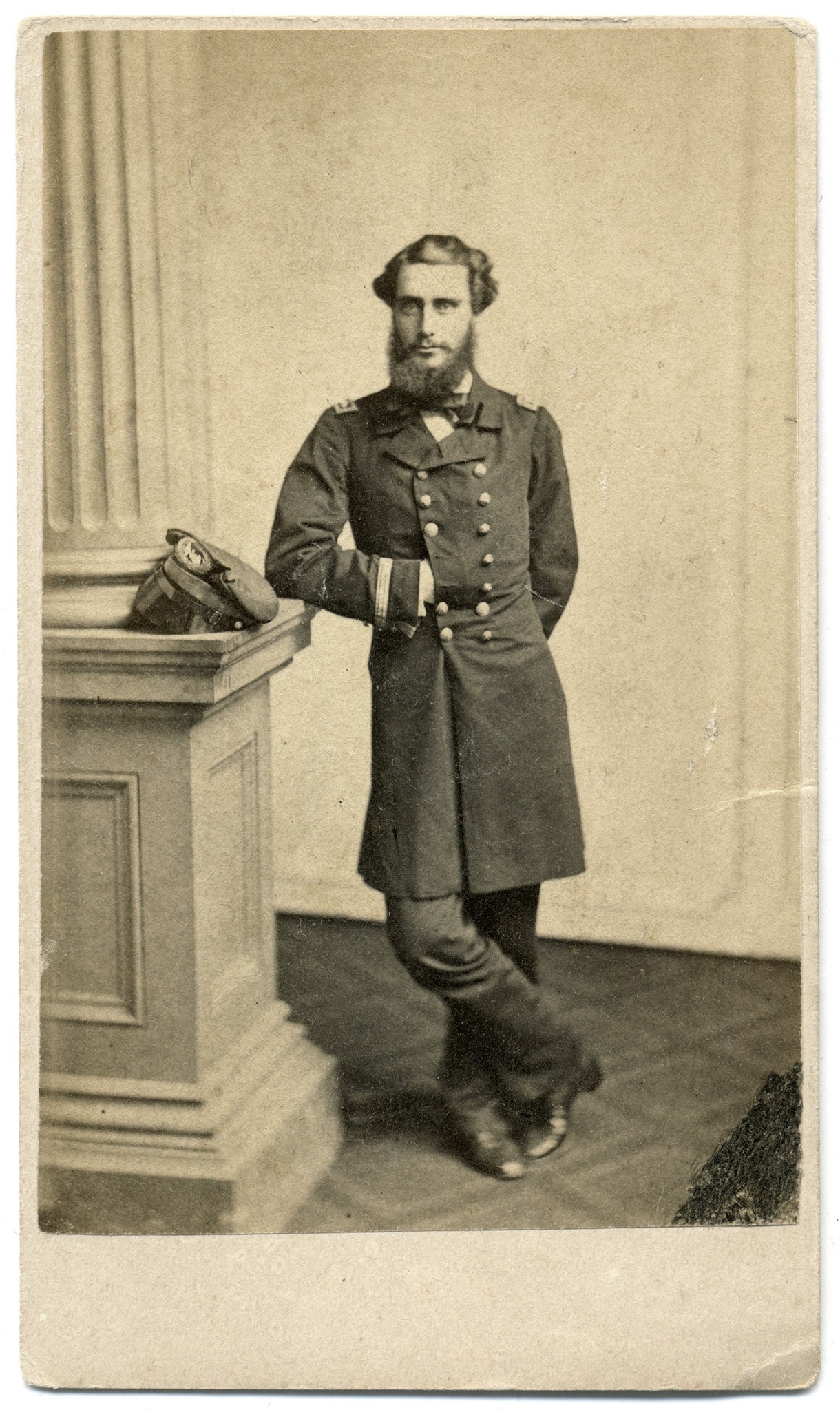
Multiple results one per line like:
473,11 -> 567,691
409,391 -> 469,423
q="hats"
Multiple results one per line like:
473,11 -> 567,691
132,529 -> 279,635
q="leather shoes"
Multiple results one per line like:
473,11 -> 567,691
443,1085 -> 526,1179
526,1052 -> 603,1159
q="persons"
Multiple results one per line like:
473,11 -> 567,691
265,232 -> 603,1180
